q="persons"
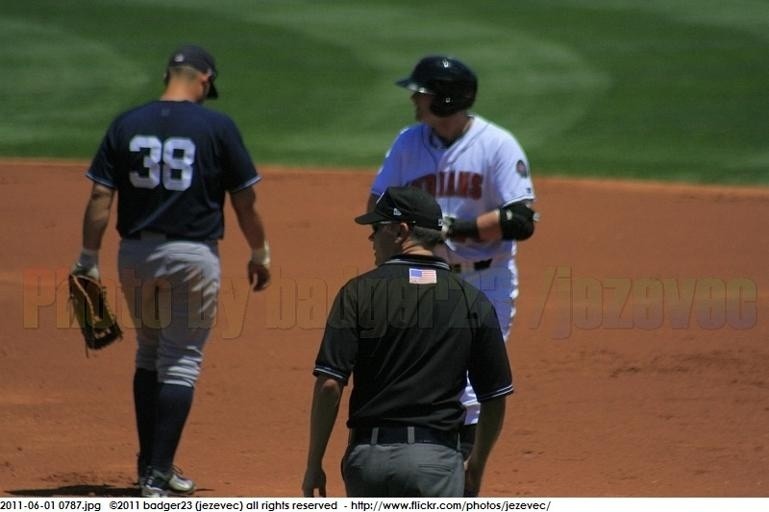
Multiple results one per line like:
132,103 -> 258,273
300,184 -> 516,497
367,54 -> 541,462
71,44 -> 272,497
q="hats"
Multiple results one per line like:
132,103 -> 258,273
353,183 -> 443,231
168,42 -> 220,102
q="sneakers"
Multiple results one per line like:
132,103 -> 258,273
136,463 -> 194,497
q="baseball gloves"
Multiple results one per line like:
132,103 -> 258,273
68,267 -> 124,359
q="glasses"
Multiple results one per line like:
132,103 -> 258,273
372,223 -> 387,233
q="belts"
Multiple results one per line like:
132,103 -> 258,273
451,258 -> 491,275
349,427 -> 459,451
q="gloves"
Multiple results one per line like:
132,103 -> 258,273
441,212 -> 457,243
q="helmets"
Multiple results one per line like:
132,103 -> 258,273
394,55 -> 479,119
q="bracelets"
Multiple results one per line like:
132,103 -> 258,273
450,218 -> 482,243
